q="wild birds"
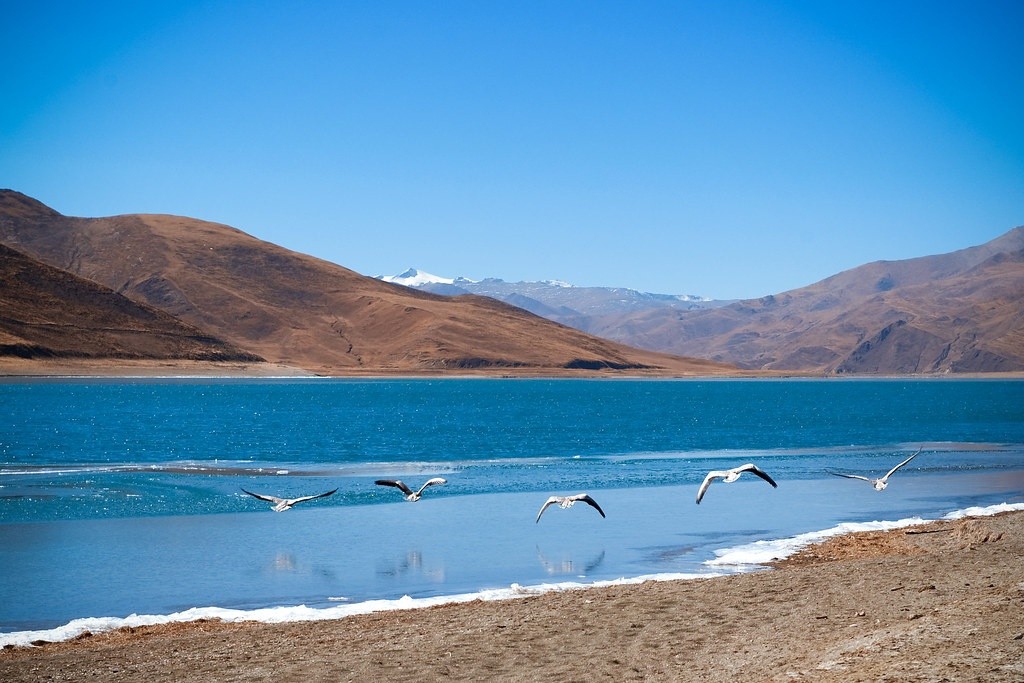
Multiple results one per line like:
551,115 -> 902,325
696,463 -> 779,505
535,493 -> 606,524
373,478 -> 447,502
824,445 -> 925,491
239,487 -> 341,512
